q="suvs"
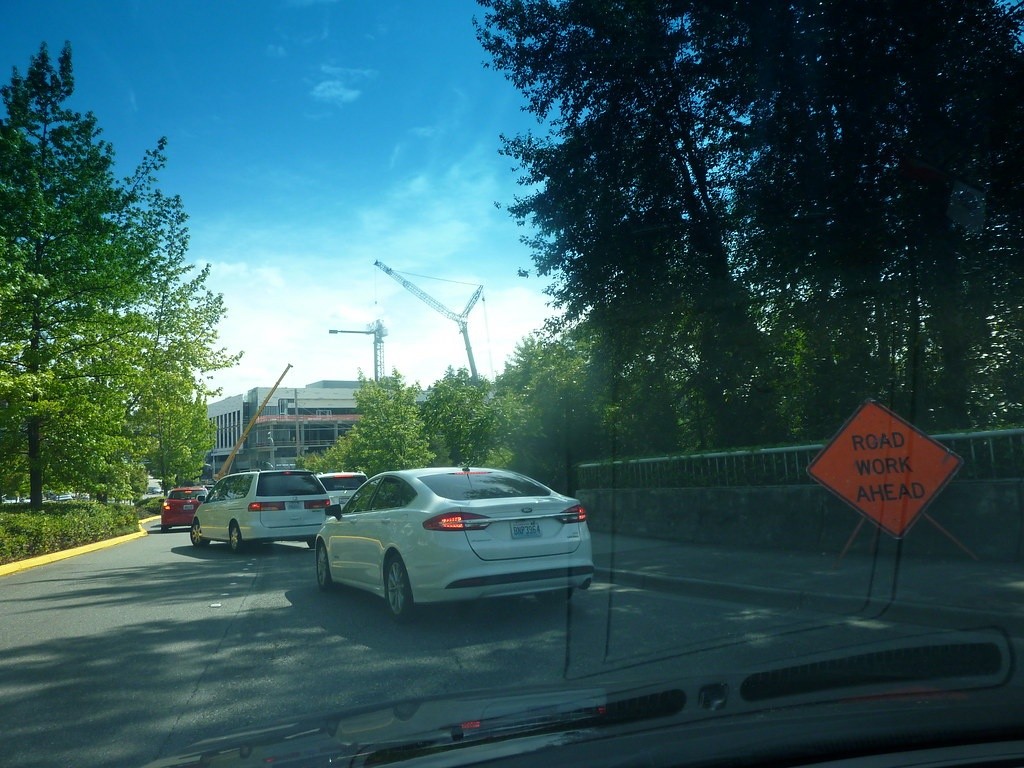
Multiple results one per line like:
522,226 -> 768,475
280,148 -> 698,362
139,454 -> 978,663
317,470 -> 368,511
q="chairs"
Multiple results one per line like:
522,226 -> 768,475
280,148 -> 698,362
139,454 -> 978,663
174,494 -> 181,499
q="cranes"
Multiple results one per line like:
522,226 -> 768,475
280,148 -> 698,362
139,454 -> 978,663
373,260 -> 485,380
329,319 -> 388,381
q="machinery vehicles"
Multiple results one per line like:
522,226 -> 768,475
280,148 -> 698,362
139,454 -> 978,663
216,363 -> 293,480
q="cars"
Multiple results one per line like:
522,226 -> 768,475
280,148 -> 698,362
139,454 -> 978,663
50,490 -> 72,501
158,487 -> 209,532
2,490 -> 44,503
189,470 -> 331,553
145,485 -> 161,494
315,467 -> 594,621
201,485 -> 218,500
